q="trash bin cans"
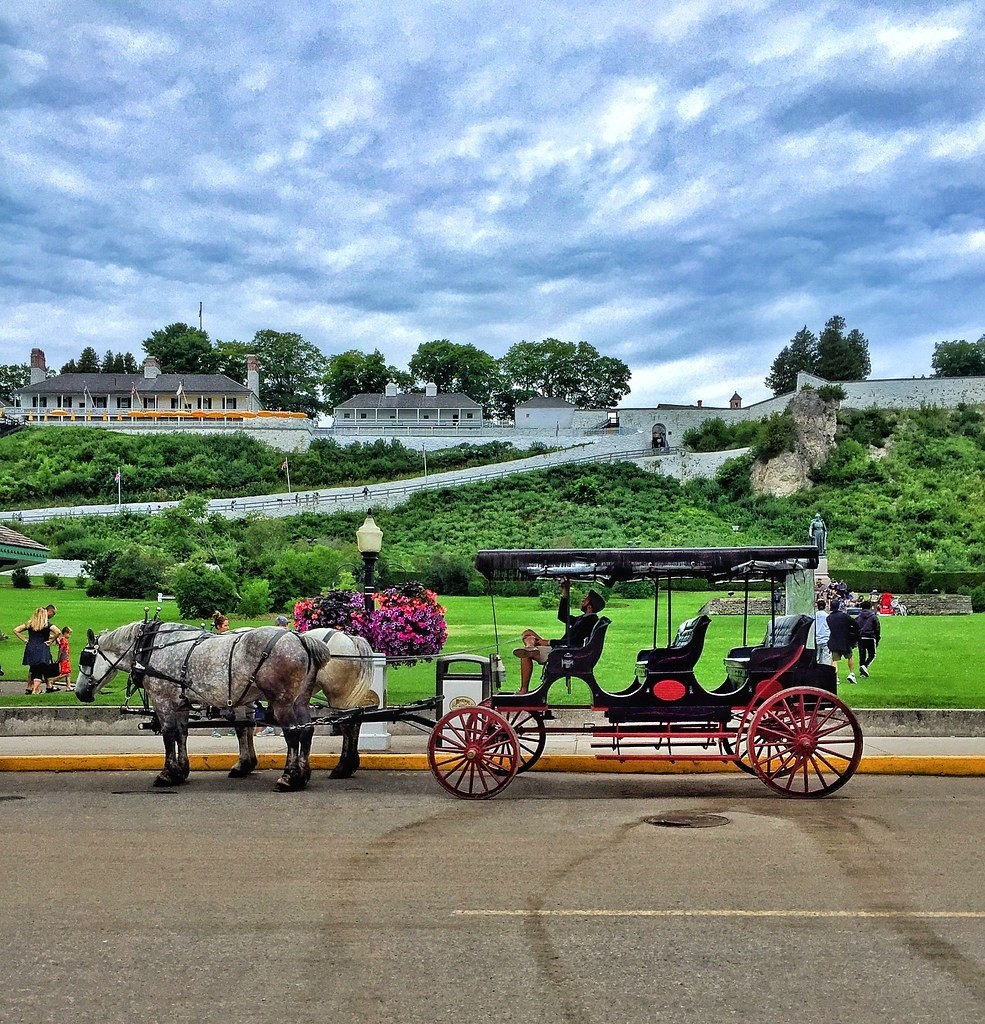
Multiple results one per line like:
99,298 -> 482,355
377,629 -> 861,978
435,655 -> 492,749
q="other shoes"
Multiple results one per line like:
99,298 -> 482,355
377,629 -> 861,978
228,729 -> 236,736
25,687 -> 42,695
66,688 -> 75,692
211,730 -> 221,738
513,647 -> 539,658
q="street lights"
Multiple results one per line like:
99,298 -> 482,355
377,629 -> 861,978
333,508 -> 409,648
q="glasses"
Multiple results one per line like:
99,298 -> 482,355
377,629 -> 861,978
52,612 -> 55,615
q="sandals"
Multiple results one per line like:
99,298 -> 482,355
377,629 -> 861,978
45,686 -> 61,692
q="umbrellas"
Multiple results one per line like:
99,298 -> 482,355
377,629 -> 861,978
29,410 -> 308,422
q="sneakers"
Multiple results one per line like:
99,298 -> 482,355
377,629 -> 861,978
256,727 -> 276,736
836,677 -> 840,684
859,665 -> 869,677
279,730 -> 284,737
847,672 -> 857,684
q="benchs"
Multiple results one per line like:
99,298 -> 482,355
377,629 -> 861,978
634,614 -> 712,677
724,614 -> 816,669
544,616 -> 611,675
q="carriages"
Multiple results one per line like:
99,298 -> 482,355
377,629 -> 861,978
74,537 -> 865,804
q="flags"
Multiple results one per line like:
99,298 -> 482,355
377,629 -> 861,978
176,385 -> 183,396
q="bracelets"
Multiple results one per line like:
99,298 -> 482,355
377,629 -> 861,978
24,641 -> 27,644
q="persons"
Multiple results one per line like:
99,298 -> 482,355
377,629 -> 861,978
12,512 -> 22,521
774,577 -> 897,615
814,600 -> 831,665
854,602 -> 880,677
13,604 -> 76,695
826,601 -> 859,685
296,492 -> 316,506
513,580 -> 605,695
210,611 -> 291,737
364,486 -> 369,500
232,500 -> 237,511
809,513 -> 827,556
147,505 -> 173,515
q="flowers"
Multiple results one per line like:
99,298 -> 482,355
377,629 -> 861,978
293,580 -> 449,670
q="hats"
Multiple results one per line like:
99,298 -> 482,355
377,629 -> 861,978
274,616 -> 291,624
817,601 -> 825,609
829,600 -> 840,612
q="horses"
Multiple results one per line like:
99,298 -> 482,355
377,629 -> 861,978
74,618 -> 331,791
218,627 -> 375,779
